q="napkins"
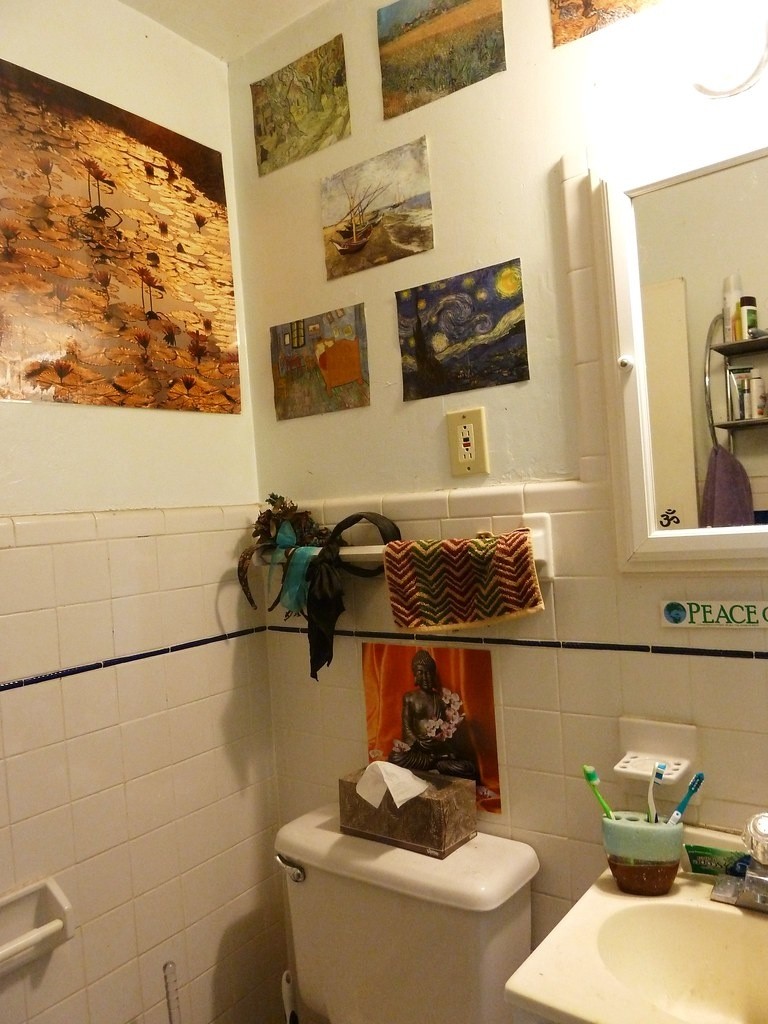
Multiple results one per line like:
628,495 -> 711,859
355,761 -> 429,809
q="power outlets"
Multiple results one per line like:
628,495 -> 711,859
445,407 -> 491,477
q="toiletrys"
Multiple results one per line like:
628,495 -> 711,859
721,267 -> 766,422
680,839 -> 757,878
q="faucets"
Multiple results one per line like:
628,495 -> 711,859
738,810 -> 768,914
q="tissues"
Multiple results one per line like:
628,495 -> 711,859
336,759 -> 482,860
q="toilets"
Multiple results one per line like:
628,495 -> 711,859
270,800 -> 540,1024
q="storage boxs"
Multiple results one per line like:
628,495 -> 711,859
339,765 -> 479,861
601,810 -> 684,895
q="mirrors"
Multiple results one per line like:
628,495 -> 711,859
601,131 -> 767,562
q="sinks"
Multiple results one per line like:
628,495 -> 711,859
500,823 -> 768,1024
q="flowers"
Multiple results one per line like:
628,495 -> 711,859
250,491 -> 333,546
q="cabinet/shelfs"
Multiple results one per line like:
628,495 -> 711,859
709,334 -> 767,430
613,716 -> 702,806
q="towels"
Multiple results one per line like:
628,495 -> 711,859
697,442 -> 756,532
382,526 -> 547,634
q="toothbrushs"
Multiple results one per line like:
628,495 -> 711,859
668,771 -> 705,828
645,759 -> 668,823
580,762 -> 619,821
